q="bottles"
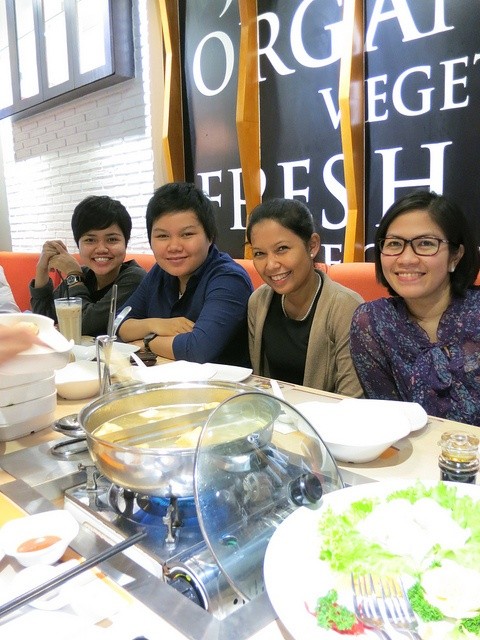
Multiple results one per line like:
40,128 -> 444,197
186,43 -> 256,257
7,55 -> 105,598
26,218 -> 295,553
437,432 -> 480,483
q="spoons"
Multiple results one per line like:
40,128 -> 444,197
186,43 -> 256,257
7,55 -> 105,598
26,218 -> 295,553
269,380 -> 296,424
51,283 -> 118,429
51,306 -> 132,438
128,350 -> 148,368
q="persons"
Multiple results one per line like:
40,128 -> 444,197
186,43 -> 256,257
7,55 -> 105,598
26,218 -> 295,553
240,198 -> 366,399
0,264 -> 22,314
349,191 -> 480,427
29,195 -> 147,337
115,181 -> 254,369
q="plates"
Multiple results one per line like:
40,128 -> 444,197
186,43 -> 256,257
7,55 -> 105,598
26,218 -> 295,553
263,479 -> 480,640
88,342 -> 141,361
74,336 -> 98,361
202,363 -> 253,382
343,400 -> 428,431
127,360 -> 217,384
0,314 -> 76,440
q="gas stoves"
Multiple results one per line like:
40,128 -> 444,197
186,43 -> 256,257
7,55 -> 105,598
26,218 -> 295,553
63,460 -> 343,612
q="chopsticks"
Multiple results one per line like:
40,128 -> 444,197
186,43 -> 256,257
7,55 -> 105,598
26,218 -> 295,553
0,531 -> 149,616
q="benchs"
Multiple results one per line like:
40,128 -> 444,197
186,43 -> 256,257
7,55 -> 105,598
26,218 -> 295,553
0,250 -> 480,313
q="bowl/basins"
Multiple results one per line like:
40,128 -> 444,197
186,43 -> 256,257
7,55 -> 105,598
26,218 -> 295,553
0,510 -> 80,567
55,360 -> 119,400
296,401 -> 412,464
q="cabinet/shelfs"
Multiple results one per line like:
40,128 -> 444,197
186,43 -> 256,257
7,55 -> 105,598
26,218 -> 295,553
1,0 -> 134,123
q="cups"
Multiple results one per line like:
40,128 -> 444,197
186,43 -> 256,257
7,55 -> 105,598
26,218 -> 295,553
54,297 -> 83,345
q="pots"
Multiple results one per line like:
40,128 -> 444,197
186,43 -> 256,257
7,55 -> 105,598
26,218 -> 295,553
79,381 -> 283,500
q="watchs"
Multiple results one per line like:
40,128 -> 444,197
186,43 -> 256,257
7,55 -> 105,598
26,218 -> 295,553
143,332 -> 157,353
65,275 -> 84,286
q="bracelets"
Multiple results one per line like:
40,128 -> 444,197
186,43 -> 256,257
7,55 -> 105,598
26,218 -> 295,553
66,270 -> 84,278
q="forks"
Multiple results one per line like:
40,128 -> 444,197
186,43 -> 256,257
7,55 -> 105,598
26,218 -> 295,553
350,573 -> 393,640
379,576 -> 423,640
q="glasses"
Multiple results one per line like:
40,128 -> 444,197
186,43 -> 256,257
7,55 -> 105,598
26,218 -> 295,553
379,237 -> 450,257
